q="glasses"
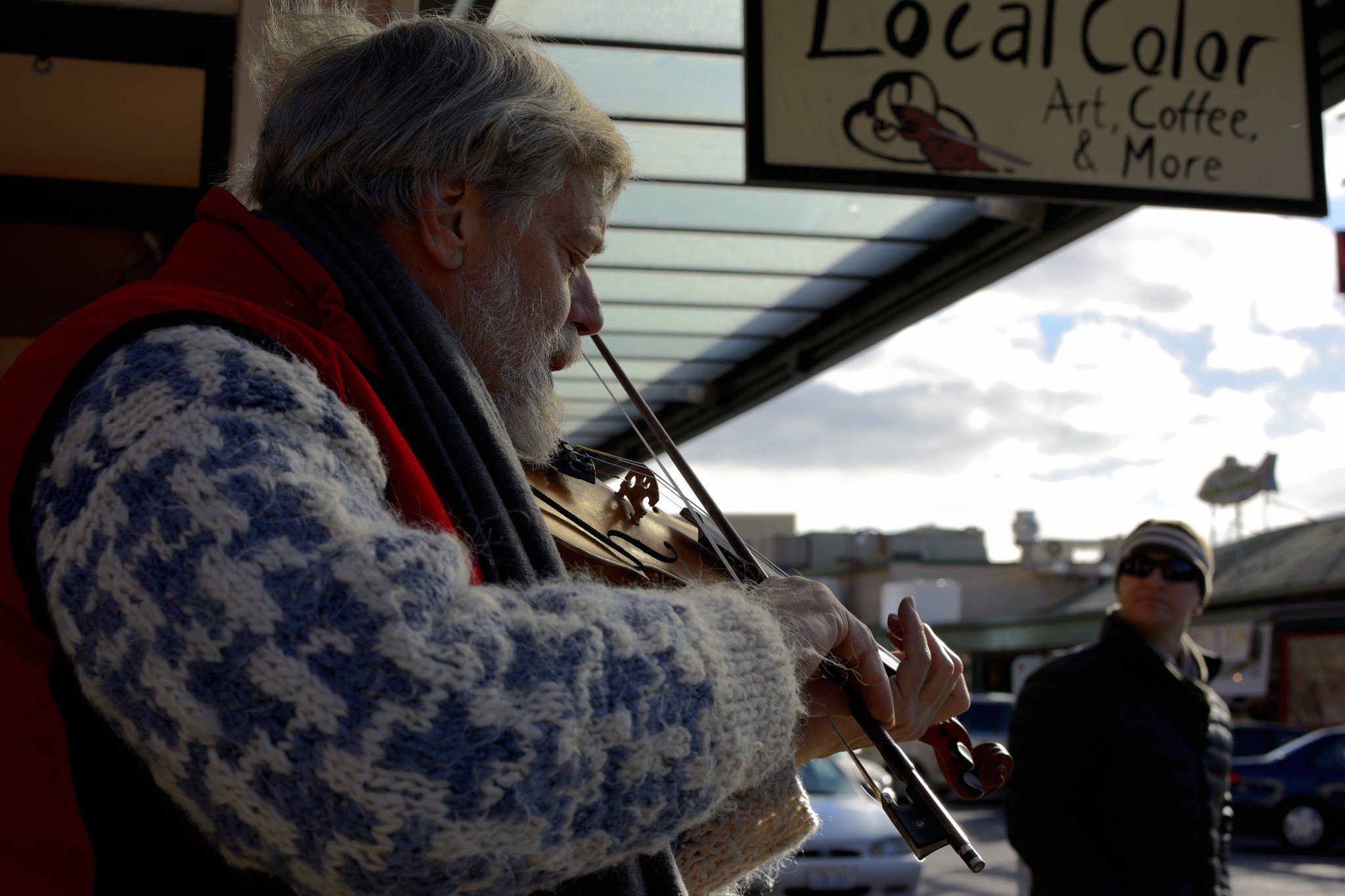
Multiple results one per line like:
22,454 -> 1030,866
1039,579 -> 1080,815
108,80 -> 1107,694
1121,556 -> 1200,584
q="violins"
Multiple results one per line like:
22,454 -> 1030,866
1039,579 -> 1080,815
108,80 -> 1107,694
514,436 -> 1017,805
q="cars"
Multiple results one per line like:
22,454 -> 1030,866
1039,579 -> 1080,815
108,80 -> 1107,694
856,691 -> 1017,794
732,756 -> 927,896
1225,721 -> 1345,859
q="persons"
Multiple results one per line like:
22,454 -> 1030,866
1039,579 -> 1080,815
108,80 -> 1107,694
0,13 -> 971,896
997,519 -> 1233,895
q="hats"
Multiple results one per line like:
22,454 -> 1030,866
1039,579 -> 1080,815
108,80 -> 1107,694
1113,519 -> 1215,610
